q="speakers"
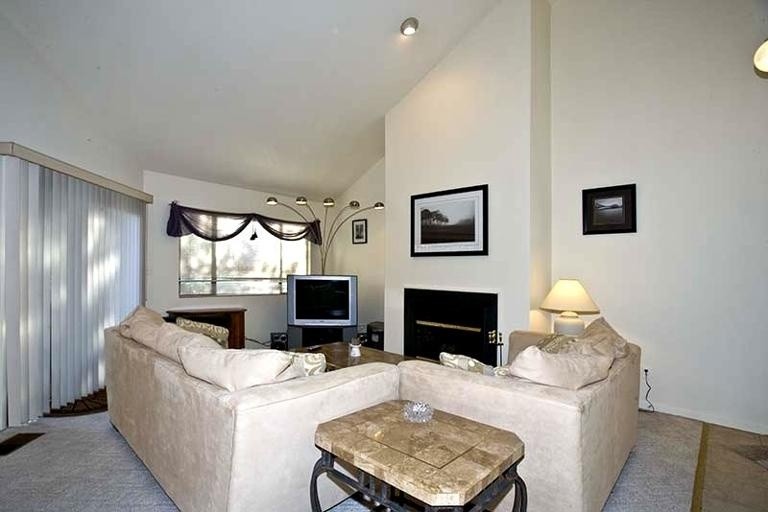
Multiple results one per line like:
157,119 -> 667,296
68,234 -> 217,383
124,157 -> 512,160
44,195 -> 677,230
367,321 -> 384,351
271,333 -> 289,351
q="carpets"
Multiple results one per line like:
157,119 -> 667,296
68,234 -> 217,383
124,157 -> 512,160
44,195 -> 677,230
0,409 -> 710,512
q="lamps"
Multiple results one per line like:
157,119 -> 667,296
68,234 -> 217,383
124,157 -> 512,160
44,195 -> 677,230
751,39 -> 768,77
266,196 -> 385,275
539,280 -> 600,335
400,17 -> 419,35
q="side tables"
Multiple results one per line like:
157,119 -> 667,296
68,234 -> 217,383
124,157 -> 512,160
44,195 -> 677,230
309,400 -> 527,512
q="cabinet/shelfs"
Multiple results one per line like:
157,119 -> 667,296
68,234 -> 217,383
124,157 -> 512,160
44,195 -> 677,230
286,326 -> 357,352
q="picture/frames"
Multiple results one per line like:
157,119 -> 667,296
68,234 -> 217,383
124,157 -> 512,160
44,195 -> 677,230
582,184 -> 636,235
352,219 -> 367,244
411,184 -> 489,256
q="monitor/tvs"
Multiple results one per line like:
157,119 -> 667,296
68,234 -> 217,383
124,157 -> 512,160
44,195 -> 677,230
287,275 -> 358,327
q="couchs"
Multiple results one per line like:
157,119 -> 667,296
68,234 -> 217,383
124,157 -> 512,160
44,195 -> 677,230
103,303 -> 642,512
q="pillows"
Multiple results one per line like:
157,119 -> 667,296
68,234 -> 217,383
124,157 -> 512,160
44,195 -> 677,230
118,307 -> 327,391
437,310 -> 631,388
